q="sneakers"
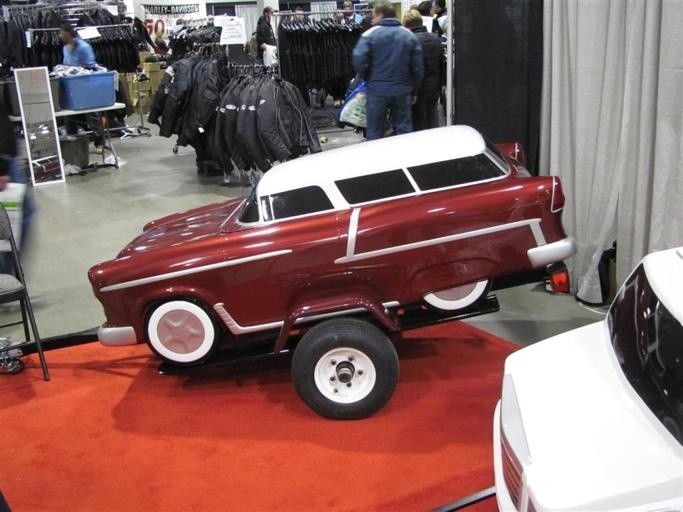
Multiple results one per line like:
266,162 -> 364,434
96,144 -> 105,155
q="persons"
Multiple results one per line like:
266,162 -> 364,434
253,0 -> 449,141
59,23 -> 106,153
0,84 -> 37,274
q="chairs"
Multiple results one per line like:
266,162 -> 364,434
0,200 -> 51,382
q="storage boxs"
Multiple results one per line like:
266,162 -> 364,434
0,180 -> 26,253
120,48 -> 168,113
0,68 -> 115,170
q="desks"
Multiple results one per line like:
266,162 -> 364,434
7,102 -> 126,169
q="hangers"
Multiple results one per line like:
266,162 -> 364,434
1,1 -> 130,47
278,8 -> 374,34
175,15 -> 279,83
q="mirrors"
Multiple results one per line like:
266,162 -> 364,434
12,64 -> 67,189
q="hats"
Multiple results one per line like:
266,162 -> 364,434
263,6 -> 278,14
